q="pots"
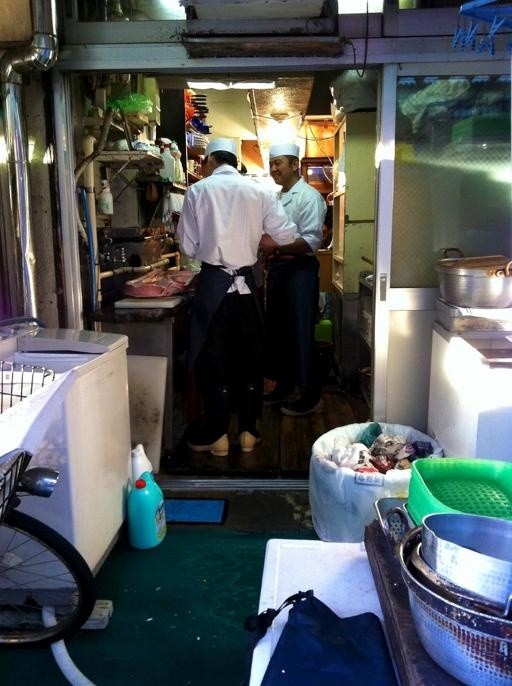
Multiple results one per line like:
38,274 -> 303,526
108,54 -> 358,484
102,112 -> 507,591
434,246 -> 512,310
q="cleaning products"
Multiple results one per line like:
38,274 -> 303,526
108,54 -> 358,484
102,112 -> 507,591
157,136 -> 175,182
126,443 -> 167,548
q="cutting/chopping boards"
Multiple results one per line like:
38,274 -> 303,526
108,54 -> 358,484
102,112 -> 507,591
114,294 -> 183,309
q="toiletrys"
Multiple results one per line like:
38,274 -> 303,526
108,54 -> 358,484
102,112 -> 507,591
98,179 -> 117,221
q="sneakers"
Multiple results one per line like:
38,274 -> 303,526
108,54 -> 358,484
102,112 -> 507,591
280,395 -> 322,416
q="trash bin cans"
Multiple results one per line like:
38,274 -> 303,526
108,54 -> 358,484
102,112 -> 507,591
308,421 -> 444,542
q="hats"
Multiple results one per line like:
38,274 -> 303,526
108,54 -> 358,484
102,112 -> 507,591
204,137 -> 238,160
269,143 -> 300,159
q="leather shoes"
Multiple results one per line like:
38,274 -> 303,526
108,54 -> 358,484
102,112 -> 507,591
237,430 -> 262,453
186,432 -> 230,458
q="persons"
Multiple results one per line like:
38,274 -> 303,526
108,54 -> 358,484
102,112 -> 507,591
257,140 -> 330,417
173,137 -> 300,458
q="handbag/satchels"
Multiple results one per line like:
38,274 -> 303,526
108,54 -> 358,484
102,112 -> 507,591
240,590 -> 398,686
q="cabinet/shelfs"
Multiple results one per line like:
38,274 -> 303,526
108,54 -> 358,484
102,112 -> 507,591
331,111 -> 379,392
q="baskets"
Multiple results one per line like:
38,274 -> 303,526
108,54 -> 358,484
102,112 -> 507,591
0,361 -> 56,525
